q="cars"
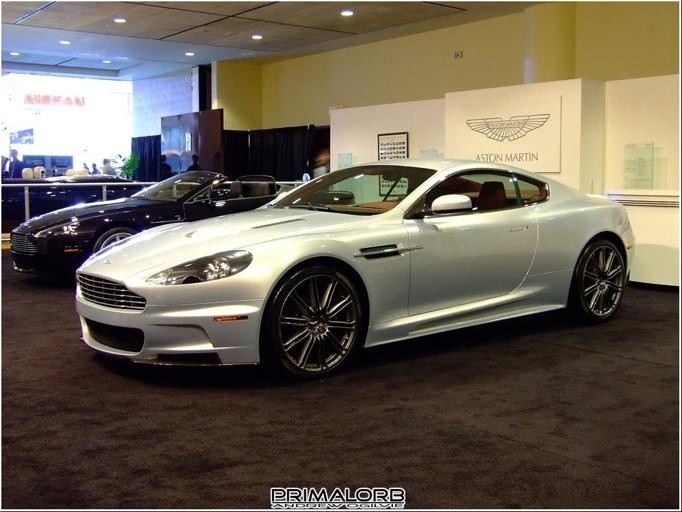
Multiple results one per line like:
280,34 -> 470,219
73,159 -> 633,382
10,171 -> 355,274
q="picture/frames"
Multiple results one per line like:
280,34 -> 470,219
377,131 -> 410,197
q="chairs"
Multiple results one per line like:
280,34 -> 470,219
477,180 -> 506,211
229,182 -> 271,197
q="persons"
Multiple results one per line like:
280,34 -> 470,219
9,149 -> 24,179
157,155 -> 171,180
187,154 -> 201,171
91,163 -> 101,174
102,158 -> 116,175
